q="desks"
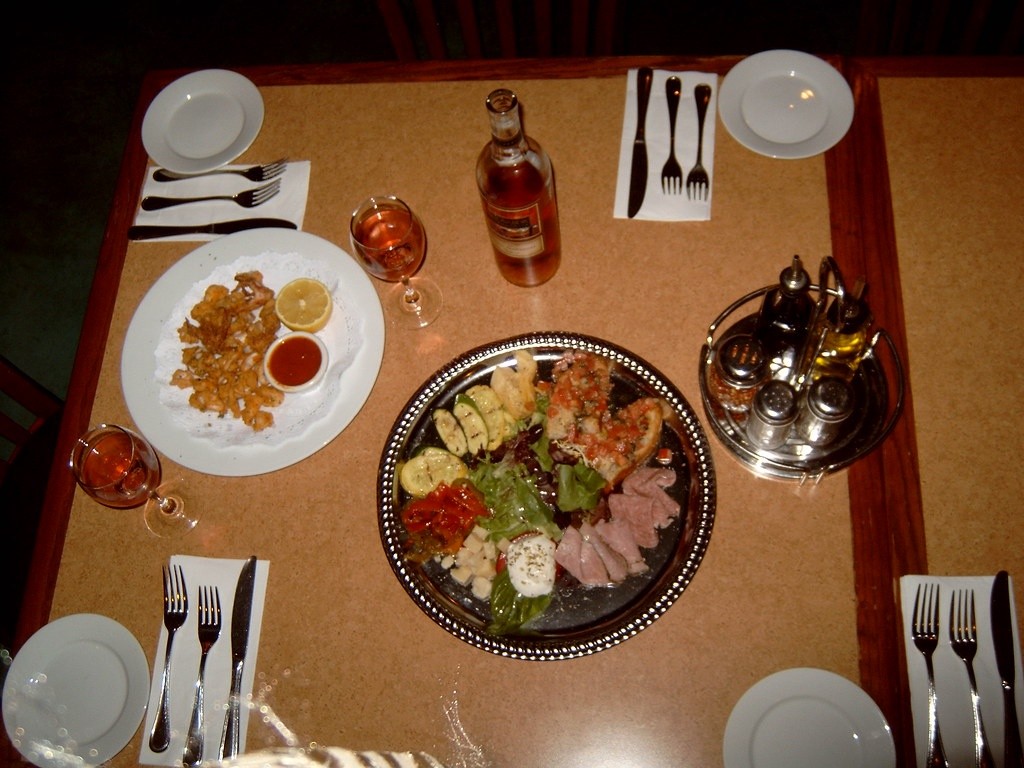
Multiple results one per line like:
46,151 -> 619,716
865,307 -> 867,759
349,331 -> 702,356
853,51 -> 1024,768
0,45 -> 903,768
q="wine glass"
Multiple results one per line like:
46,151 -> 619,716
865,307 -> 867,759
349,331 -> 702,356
67,424 -> 204,539
348,195 -> 444,329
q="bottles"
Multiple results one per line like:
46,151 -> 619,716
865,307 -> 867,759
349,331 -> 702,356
475,89 -> 562,288
710,253 -> 871,450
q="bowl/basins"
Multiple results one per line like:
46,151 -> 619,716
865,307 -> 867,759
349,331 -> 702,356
263,332 -> 329,394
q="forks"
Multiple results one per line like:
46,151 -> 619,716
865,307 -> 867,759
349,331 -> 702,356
660,75 -> 712,202
149,564 -> 222,768
910,581 -> 997,768
140,155 -> 290,208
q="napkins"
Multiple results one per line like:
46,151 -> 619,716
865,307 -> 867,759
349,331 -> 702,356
615,64 -> 718,221
139,556 -> 273,767
134,159 -> 313,246
896,574 -> 1024,768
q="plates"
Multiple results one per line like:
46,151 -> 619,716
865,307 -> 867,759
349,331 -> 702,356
722,666 -> 897,768
376,331 -> 718,662
121,227 -> 385,477
2,613 -> 153,768
141,69 -> 264,175
718,48 -> 855,159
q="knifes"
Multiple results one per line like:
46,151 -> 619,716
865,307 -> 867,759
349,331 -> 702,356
128,218 -> 298,240
627,65 -> 652,218
219,554 -> 258,759
990,569 -> 1024,768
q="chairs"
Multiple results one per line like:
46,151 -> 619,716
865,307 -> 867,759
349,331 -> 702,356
0,354 -> 64,646
379,1 -> 620,60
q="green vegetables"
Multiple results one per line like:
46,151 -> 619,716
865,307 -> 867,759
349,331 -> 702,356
476,394 -> 611,635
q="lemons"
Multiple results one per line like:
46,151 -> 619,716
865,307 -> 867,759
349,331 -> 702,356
274,277 -> 332,335
